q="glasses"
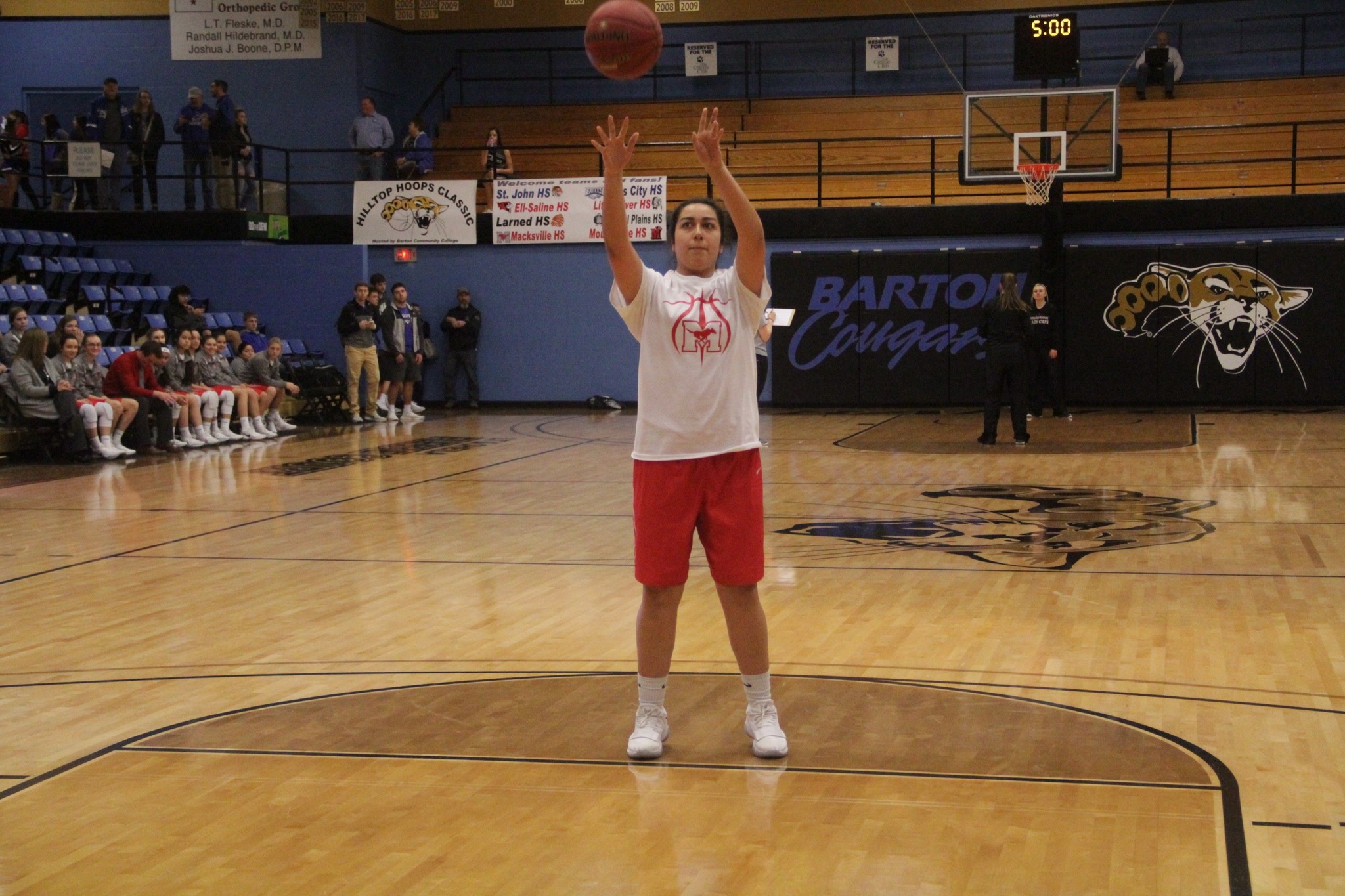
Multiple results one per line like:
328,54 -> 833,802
84,343 -> 103,347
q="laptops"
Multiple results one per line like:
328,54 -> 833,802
1145,48 -> 1169,67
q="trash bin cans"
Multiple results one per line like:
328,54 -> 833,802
256,182 -> 287,216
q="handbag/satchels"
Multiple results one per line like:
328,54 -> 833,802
423,339 -> 440,360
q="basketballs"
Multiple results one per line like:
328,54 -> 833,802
584,0 -> 662,80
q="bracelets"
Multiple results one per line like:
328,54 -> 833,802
768,318 -> 774,322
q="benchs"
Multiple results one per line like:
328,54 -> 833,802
422,75 -> 1344,212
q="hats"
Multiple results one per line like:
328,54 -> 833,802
188,86 -> 203,98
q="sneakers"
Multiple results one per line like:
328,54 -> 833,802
180,435 -> 296,461
376,420 -> 425,432
351,414 -> 363,423
744,699 -> 790,758
744,756 -> 788,798
365,413 -> 387,422
375,393 -> 425,421
627,703 -> 670,758
629,759 -> 669,793
164,414 -> 296,446
87,459 -> 137,482
92,443 -> 137,459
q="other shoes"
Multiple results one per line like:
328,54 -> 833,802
1015,439 -> 1025,444
1027,413 -> 1043,418
141,446 -> 166,456
157,442 -> 184,453
1055,413 -> 1073,418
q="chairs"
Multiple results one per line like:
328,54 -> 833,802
0,226 -> 350,429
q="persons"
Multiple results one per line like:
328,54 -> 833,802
755,305 -> 776,446
438,287 -> 482,411
480,127 -> 513,215
1025,282 -> 1075,420
1,242 -> 27,286
590,103 -> 798,760
1134,30 -> 1185,100
0,75 -> 256,209
337,274 -> 436,424
347,96 -> 392,182
977,273 -> 1038,446
0,284 -> 300,462
392,118 -> 433,179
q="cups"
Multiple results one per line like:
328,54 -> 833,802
245,146 -> 252,152
489,138 -> 495,147
397,159 -> 403,169
